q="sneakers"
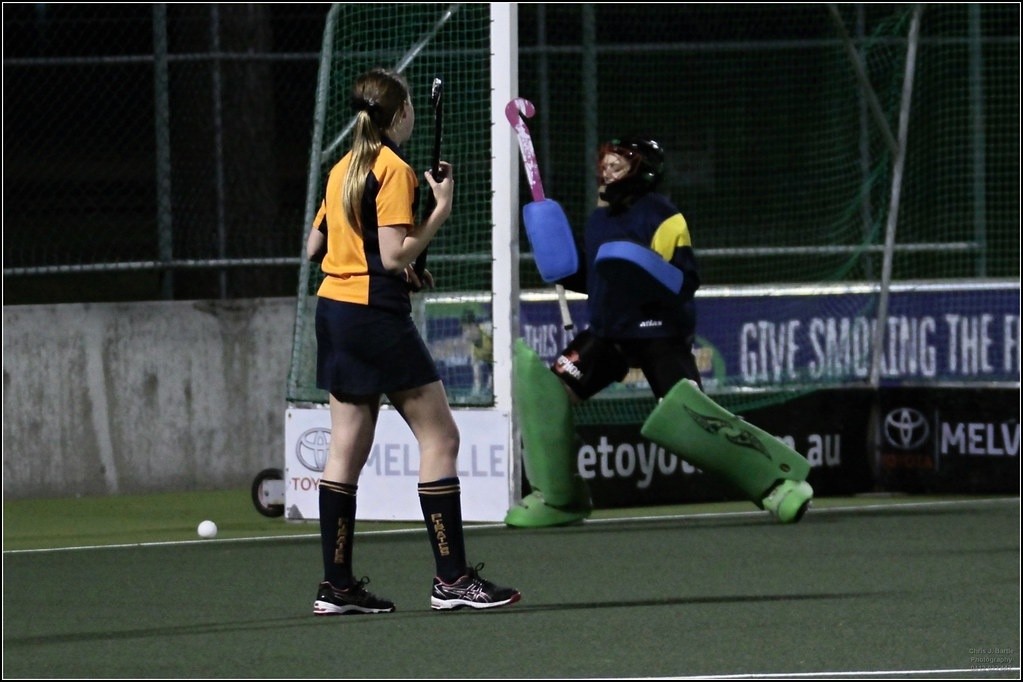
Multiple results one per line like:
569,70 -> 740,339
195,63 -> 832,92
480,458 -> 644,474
430,567 -> 522,610
312,581 -> 397,614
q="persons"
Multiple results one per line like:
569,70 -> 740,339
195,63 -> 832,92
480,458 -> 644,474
504,130 -> 815,529
302,66 -> 524,621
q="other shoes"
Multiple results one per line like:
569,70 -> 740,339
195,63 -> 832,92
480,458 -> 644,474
763,477 -> 815,525
504,495 -> 585,529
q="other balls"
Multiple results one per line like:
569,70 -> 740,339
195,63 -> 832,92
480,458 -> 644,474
197,520 -> 218,539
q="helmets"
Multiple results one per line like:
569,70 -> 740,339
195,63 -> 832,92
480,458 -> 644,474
599,135 -> 667,206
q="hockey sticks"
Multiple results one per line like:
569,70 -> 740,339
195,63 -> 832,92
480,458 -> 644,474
505,97 -> 578,352
408,78 -> 443,293
